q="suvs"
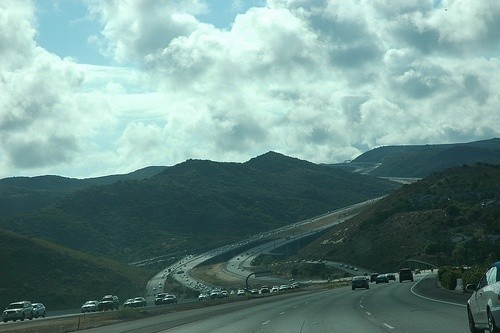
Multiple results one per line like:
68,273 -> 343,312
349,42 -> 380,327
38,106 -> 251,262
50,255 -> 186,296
2,301 -> 33,323
371,272 -> 378,282
97,295 -> 119,312
398,268 -> 414,283
30,302 -> 46,318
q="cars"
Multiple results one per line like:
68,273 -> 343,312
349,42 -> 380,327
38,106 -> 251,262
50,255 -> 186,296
131,297 -> 146,308
387,273 -> 396,281
465,262 -> 500,333
152,230 -> 369,303
81,300 -> 99,313
163,294 -> 177,304
122,299 -> 133,308
415,268 -> 420,275
155,293 -> 164,305
352,275 -> 370,290
376,274 -> 389,283
